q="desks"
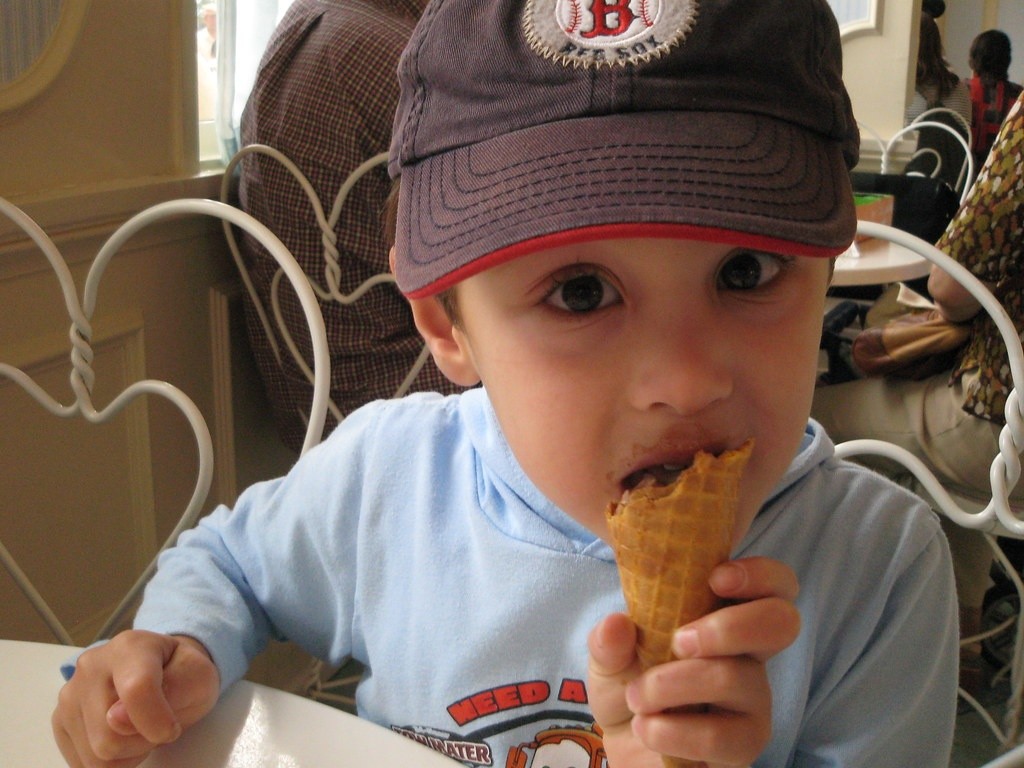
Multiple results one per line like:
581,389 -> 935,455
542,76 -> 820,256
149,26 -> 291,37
0,638 -> 472,768
827,233 -> 930,387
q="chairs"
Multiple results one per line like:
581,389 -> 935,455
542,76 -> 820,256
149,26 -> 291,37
1,107 -> 1024,768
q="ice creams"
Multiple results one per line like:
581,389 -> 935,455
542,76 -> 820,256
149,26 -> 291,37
606,437 -> 755,767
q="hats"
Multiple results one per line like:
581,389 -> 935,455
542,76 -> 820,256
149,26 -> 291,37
386,1 -> 858,298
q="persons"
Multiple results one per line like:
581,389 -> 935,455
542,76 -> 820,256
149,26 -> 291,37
53,0 -> 959,768
811,0 -> 1024,507
